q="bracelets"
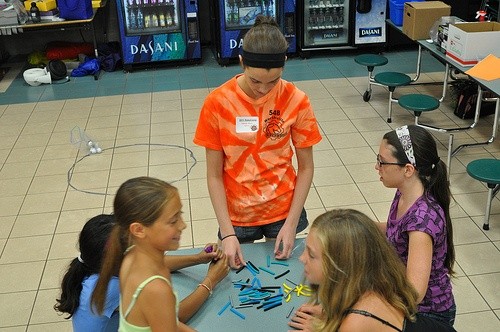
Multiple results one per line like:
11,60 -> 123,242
197,284 -> 211,294
221,234 -> 236,241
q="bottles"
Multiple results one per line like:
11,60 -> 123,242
308,0 -> 343,27
126,0 -> 175,30
227,0 -> 273,25
30,2 -> 41,23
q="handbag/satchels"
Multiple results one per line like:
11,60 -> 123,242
70,53 -> 99,77
98,48 -> 122,72
23,59 -> 70,86
58,0 -> 94,19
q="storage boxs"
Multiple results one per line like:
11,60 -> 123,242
402,2 -> 451,41
23,0 -> 103,20
446,21 -> 500,66
436,25 -> 450,54
387,0 -> 426,27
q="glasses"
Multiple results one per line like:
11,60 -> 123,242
376,153 -> 417,170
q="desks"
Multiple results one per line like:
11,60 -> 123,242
386,19 -> 500,144
166,238 -> 319,332
0,5 -> 108,79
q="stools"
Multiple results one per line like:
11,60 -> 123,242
355,54 -> 388,101
466,158 -> 499,230
397,94 -> 440,127
375,72 -> 410,124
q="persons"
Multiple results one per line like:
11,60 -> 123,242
55,213 -> 230,332
367,124 -> 456,332
90,176 -> 200,332
288,208 -> 418,332
192,15 -> 323,270
